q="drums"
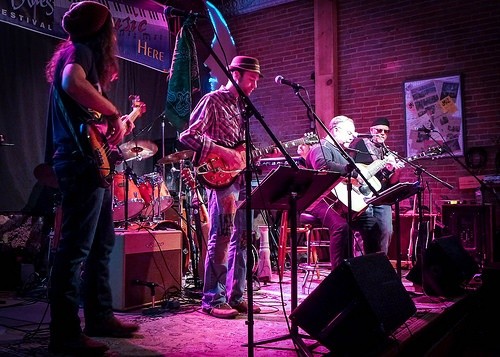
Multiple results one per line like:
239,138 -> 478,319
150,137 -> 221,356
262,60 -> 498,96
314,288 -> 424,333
136,171 -> 174,217
434,198 -> 477,250
105,171 -> 146,223
115,220 -> 191,277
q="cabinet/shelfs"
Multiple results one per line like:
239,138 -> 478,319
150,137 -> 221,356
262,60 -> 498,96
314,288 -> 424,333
440,200 -> 495,267
109,228 -> 183,311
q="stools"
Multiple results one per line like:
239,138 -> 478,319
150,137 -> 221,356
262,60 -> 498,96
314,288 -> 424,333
298,214 -> 329,284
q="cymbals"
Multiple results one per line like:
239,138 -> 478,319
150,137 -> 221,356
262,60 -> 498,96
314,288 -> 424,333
157,149 -> 194,164
117,139 -> 159,163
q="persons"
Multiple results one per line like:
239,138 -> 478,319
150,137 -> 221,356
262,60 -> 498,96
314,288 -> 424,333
179,55 -> 281,318
21,163 -> 62,286
305,115 -> 388,271
45,2 -> 140,357
354,117 -> 405,253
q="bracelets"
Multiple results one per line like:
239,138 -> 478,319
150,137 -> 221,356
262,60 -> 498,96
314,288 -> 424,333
105,113 -> 122,120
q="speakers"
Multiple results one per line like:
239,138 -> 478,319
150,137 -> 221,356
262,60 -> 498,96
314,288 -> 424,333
288,251 -> 416,357
108,230 -> 182,311
406,235 -> 477,298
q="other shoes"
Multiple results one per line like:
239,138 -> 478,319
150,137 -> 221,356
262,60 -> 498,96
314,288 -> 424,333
232,302 -> 261,313
57,338 -> 109,356
211,305 -> 238,318
83,316 -> 138,337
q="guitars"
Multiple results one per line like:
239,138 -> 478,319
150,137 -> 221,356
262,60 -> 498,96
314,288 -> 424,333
323,150 -> 399,220
180,167 -> 259,284
79,94 -> 147,189
194,131 -> 320,191
374,145 -> 451,183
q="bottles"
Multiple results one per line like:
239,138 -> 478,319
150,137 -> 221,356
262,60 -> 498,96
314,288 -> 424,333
482,254 -> 488,269
476,188 -> 482,204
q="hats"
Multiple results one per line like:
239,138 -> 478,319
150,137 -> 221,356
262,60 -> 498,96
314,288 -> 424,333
62,0 -> 108,39
369,118 -> 390,132
225,55 -> 264,78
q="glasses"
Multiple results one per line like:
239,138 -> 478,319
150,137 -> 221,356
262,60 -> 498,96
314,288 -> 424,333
373,128 -> 389,134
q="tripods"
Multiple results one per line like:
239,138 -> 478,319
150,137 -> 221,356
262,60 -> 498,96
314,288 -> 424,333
239,164 -> 341,356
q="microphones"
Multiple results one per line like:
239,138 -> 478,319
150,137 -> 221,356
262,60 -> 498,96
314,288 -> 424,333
163,6 -> 205,19
172,202 -> 198,209
415,127 -> 438,133
353,132 -> 381,137
274,75 -> 304,90
